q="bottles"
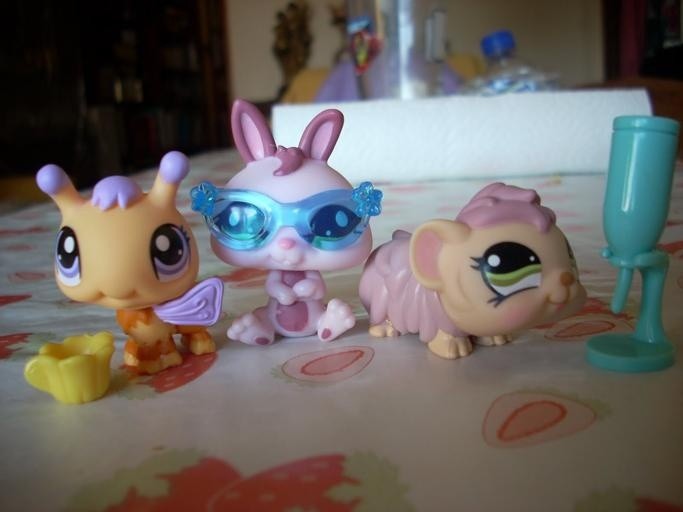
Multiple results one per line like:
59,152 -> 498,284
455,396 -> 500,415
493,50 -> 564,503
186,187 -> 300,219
480,30 -> 547,95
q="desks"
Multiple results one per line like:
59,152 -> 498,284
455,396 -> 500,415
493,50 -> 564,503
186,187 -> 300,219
0,146 -> 682,510
